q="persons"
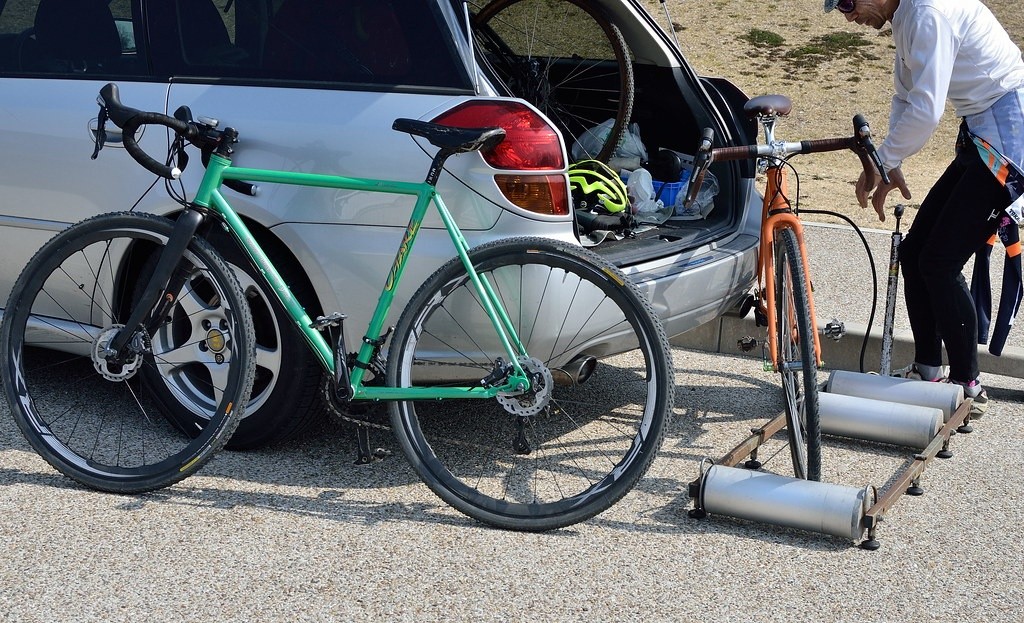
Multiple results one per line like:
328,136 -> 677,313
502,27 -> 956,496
824,0 -> 1024,421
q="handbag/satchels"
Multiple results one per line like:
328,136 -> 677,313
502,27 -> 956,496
647,151 -> 684,183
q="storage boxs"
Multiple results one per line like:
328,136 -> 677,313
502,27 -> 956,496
619,177 -> 686,208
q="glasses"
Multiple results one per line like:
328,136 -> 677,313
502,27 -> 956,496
835,0 -> 856,14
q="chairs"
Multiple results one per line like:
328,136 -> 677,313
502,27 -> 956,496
131,0 -> 231,80
33,0 -> 123,78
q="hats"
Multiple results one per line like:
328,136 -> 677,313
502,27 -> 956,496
824,0 -> 839,13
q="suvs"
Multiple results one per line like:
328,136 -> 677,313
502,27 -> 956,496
0,2 -> 776,461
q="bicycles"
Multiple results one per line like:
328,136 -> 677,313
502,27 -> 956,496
681,93 -> 892,481
1,82 -> 678,537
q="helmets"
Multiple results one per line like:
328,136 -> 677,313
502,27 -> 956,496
567,159 -> 628,215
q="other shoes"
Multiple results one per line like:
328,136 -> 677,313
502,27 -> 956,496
870,363 -> 948,384
939,376 -> 987,421
